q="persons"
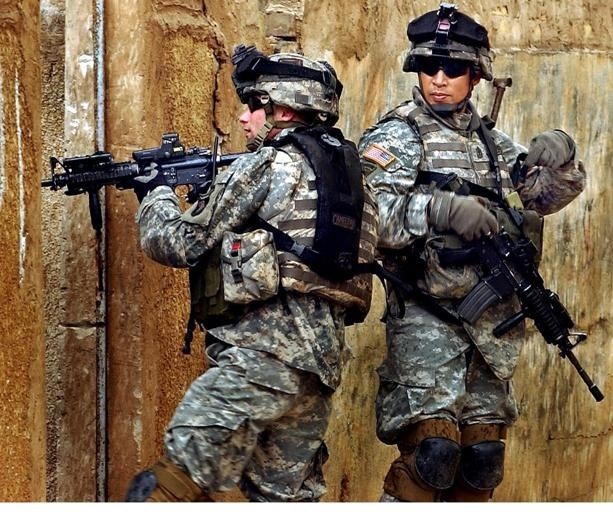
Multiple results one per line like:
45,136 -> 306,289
356,7 -> 587,501
130,51 -> 373,502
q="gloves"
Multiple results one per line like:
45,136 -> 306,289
522,129 -> 575,170
430,188 -> 500,241
133,162 -> 167,204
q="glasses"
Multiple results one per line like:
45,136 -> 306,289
415,58 -> 469,78
246,96 -> 266,113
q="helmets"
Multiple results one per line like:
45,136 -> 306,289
231,44 -> 339,128
403,5 -> 495,81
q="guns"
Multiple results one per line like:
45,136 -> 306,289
435,172 -> 605,401
40,133 -> 252,231
